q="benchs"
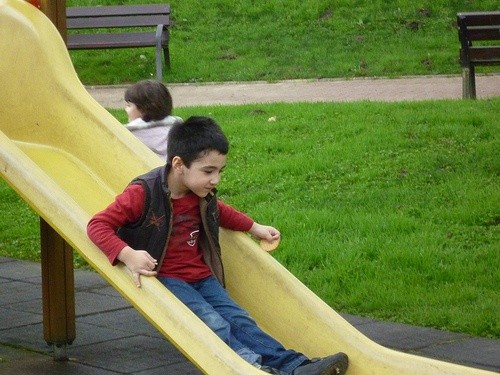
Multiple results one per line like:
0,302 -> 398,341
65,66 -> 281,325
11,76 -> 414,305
456,11 -> 500,100
65,3 -> 171,82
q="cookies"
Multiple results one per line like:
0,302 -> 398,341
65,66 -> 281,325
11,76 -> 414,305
259,236 -> 280,251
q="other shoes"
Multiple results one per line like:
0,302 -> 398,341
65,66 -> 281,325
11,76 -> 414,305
294,352 -> 349,375
260,365 -> 287,375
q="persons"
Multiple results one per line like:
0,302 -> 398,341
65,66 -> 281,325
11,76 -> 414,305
123,79 -> 184,163
86,116 -> 349,375
